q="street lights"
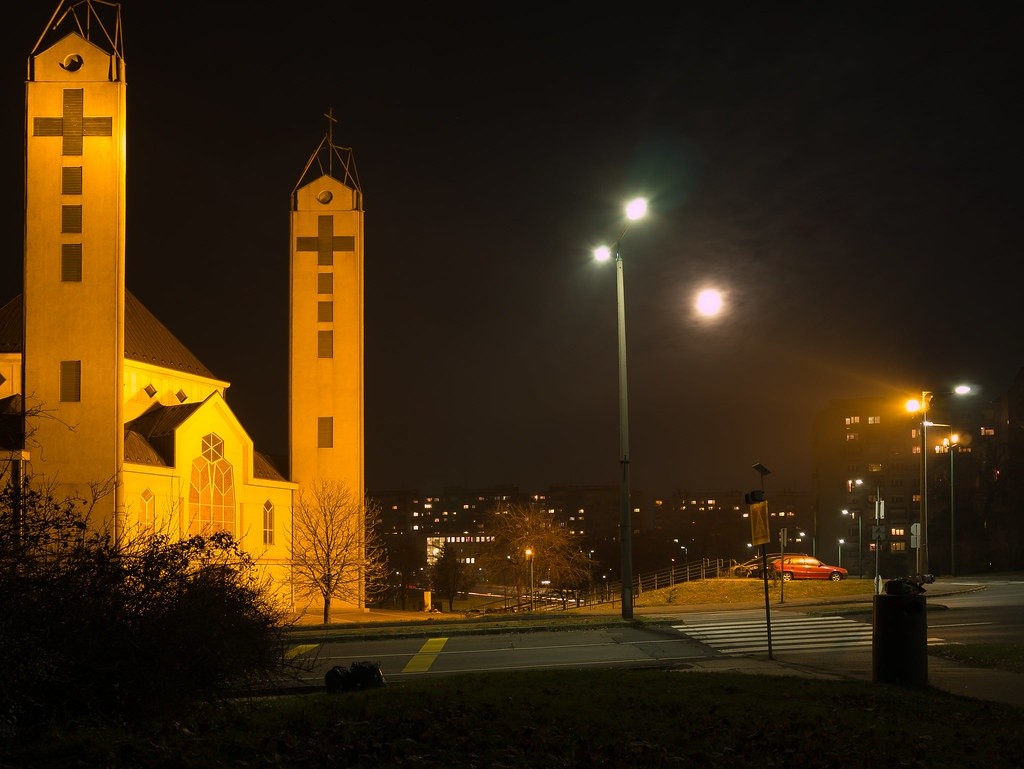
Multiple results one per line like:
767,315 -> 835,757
589,550 -> 595,594
906,384 -> 971,581
680,545 -> 687,566
526,546 -> 533,611
745,464 -> 772,657
594,197 -> 647,620
856,478 -> 881,593
839,539 -> 844,568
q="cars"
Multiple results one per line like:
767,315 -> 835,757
735,553 -> 848,581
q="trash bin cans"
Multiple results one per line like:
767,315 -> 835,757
872,595 -> 928,688
